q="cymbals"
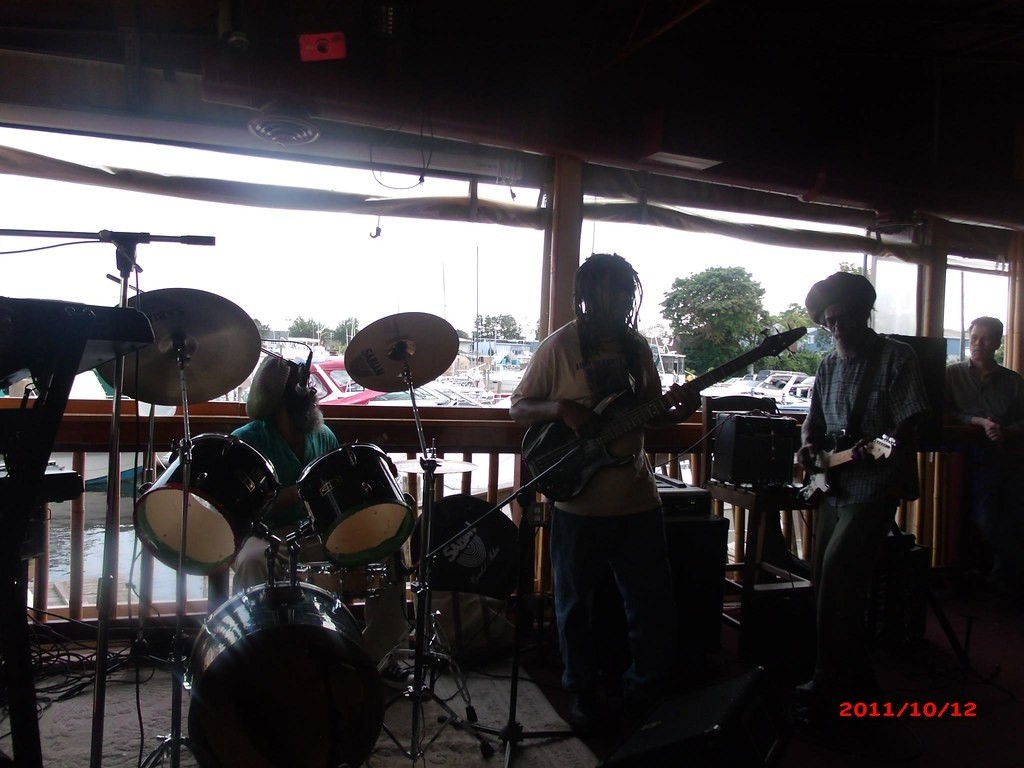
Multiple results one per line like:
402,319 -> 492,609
95,286 -> 261,408
394,458 -> 481,474
344,311 -> 461,395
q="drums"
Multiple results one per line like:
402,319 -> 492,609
292,560 -> 393,598
296,441 -> 415,563
133,431 -> 284,577
187,580 -> 384,768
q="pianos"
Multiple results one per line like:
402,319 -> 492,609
0,295 -> 157,768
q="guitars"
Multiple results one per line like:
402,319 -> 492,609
799,427 -> 902,504
521,322 -> 809,504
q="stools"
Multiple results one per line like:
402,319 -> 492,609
699,397 -> 822,629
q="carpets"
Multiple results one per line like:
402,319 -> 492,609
0,647 -> 603,768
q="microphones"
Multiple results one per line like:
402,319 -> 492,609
295,352 -> 313,394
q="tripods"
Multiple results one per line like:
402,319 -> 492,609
373,353 -> 584,768
58,274 -> 299,768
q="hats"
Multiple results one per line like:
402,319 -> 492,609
809,272 -> 876,325
244,358 -> 291,418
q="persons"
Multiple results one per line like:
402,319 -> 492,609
232,355 -> 417,692
791,273 -> 933,703
511,254 -> 700,717
946,317 -> 1024,606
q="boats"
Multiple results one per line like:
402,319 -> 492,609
0,245 -> 535,522
642,329 -> 819,412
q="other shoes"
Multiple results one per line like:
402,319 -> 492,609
795,680 -> 815,691
994,583 -> 1024,616
570,682 -> 597,721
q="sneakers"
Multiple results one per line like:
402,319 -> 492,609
375,654 -> 411,690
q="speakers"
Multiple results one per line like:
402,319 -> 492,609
594,665 -> 793,768
712,413 -> 797,484
